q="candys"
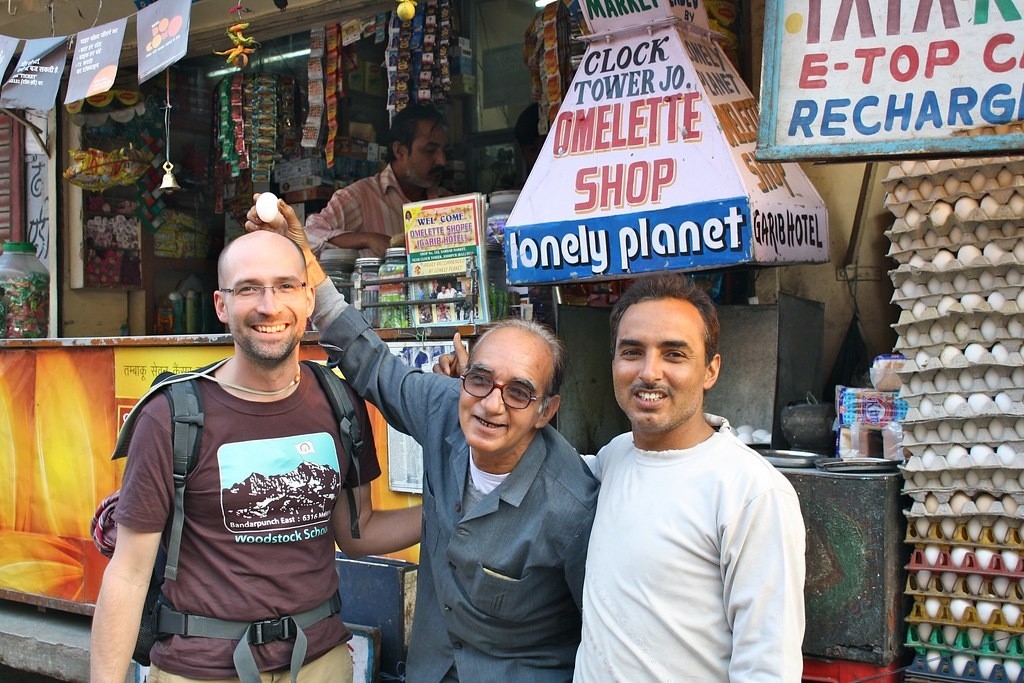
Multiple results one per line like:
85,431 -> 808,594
0,270 -> 50,338
62,142 -> 154,192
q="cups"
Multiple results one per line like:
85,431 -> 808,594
736,425 -> 772,445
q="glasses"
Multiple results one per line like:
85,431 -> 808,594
460,373 -> 549,410
220,282 -> 306,302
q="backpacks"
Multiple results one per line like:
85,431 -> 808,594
129,359 -> 363,683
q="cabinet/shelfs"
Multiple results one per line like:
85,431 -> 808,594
224,57 -> 391,241
67,44 -> 224,333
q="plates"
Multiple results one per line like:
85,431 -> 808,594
744,441 -> 906,472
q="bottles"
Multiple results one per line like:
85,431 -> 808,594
0,241 -> 52,338
155,272 -> 223,335
318,247 -> 411,329
486,190 -> 524,249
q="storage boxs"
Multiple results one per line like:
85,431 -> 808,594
777,465 -> 916,664
153,209 -> 209,257
275,122 -> 393,193
802,658 -> 913,683
348,61 -> 389,98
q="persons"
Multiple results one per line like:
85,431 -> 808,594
516,103 -> 551,172
89,229 -> 421,683
245,193 -> 601,683
301,105 -> 456,258
433,272 -> 807,683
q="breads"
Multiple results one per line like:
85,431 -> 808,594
834,353 -> 904,460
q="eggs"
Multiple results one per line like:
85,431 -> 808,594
731,424 -> 771,444
256,192 -> 279,222
893,125 -> 1024,683
